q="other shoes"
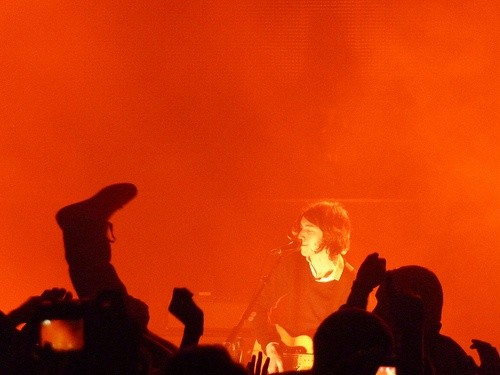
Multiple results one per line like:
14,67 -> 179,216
56,182 -> 136,241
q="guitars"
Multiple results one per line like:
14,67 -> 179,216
239,323 -> 317,375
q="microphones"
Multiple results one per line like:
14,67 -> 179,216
270,240 -> 300,256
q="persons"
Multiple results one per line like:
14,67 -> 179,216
345,252 -> 500,375
251,201 -> 357,375
0,183 -> 279,375
269,308 -> 395,375
